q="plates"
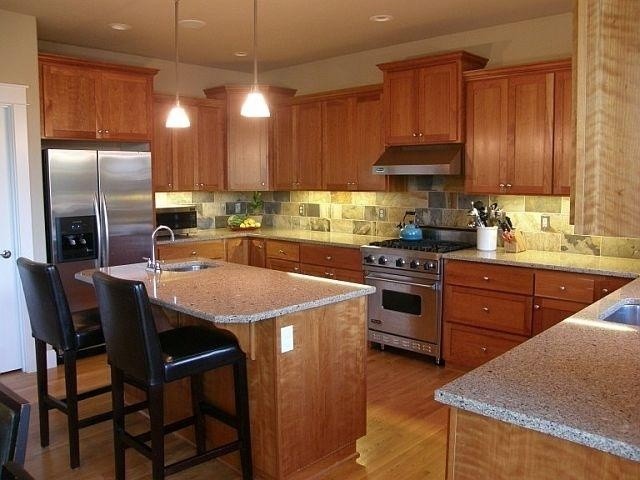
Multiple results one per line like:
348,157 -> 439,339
228,226 -> 258,231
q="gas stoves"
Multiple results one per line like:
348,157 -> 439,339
360,235 -> 475,273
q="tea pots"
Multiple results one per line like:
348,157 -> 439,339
396,210 -> 424,240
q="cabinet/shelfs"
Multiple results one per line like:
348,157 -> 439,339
463,61 -> 554,196
321,82 -> 386,191
154,238 -> 365,286
270,92 -> 322,192
152,93 -> 226,192
554,57 -> 574,195
442,256 -> 636,373
203,85 -> 298,190
375,52 -> 490,146
37,53 -> 161,141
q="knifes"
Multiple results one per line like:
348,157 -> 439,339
498,216 -> 514,235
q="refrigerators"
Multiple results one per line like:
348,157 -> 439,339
42,148 -> 156,367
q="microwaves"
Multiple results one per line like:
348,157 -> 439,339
155,205 -> 197,238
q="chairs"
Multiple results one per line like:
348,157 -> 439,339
0,382 -> 36,480
93,275 -> 255,480
16,258 -> 147,470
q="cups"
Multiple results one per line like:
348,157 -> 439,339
475,226 -> 498,251
476,249 -> 497,261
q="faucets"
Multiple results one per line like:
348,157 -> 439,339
142,224 -> 176,273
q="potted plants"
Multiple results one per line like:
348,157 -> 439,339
246,191 -> 265,227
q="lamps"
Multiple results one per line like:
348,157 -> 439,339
239,0 -> 271,119
165,0 -> 192,129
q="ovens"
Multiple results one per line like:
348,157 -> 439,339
361,271 -> 442,365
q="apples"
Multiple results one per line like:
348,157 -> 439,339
240,218 -> 261,228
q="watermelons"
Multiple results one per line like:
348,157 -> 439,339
227,215 -> 243,227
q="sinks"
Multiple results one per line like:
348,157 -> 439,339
597,297 -> 640,328
157,259 -> 222,273
